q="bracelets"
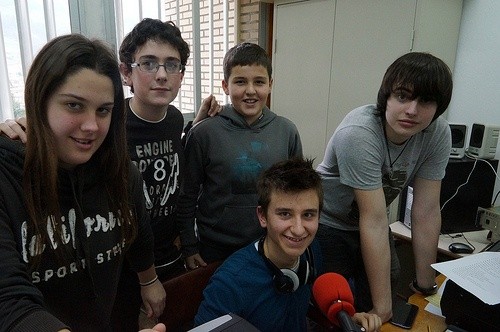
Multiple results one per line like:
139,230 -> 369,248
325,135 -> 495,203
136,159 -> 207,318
139,275 -> 157,285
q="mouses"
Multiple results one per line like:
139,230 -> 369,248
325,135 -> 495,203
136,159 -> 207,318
448,243 -> 473,254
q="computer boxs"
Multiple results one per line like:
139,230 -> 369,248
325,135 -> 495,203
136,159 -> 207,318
399,155 -> 498,235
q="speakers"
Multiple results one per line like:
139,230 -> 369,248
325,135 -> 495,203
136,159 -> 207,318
448,123 -> 468,158
467,124 -> 500,160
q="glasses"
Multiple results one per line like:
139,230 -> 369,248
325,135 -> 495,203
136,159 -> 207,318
128,60 -> 185,75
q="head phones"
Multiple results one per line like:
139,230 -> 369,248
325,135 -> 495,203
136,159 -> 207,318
258,231 -> 314,295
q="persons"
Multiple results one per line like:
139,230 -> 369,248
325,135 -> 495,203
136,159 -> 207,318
180,42 -> 303,270
1,35 -> 166,332
306,52 -> 452,324
0,17 -> 223,332
193,158 -> 383,332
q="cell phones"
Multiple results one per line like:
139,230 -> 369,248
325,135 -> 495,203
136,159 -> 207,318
389,302 -> 419,329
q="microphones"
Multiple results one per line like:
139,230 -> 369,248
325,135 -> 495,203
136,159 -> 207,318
312,272 -> 356,332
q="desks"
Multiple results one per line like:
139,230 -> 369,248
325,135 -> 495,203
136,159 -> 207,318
380,220 -> 490,332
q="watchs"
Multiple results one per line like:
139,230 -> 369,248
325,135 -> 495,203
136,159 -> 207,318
413,281 -> 438,296
